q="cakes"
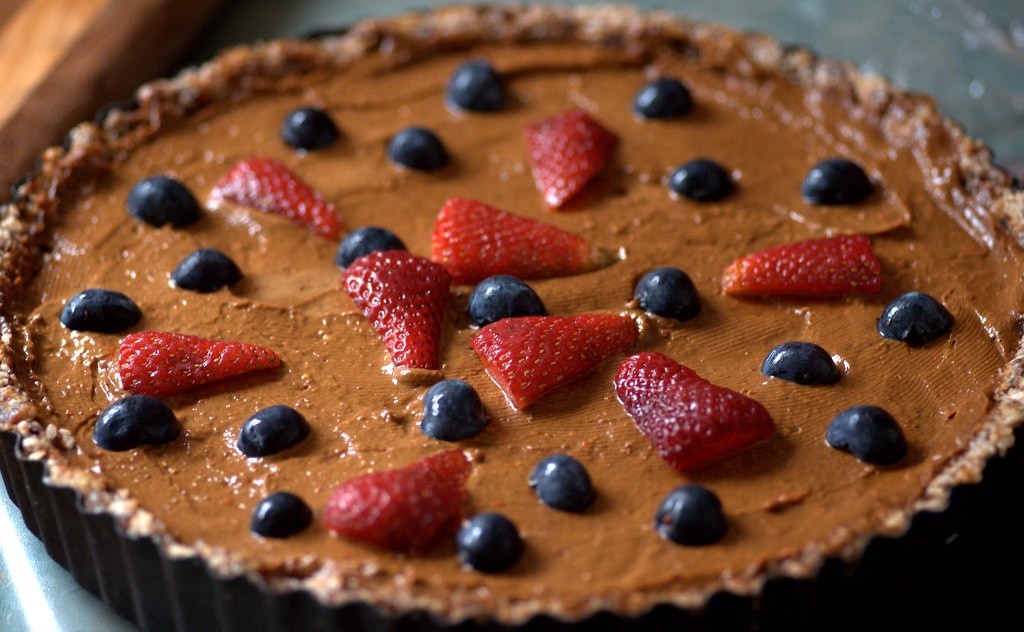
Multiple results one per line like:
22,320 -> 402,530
0,4 -> 1024,632
